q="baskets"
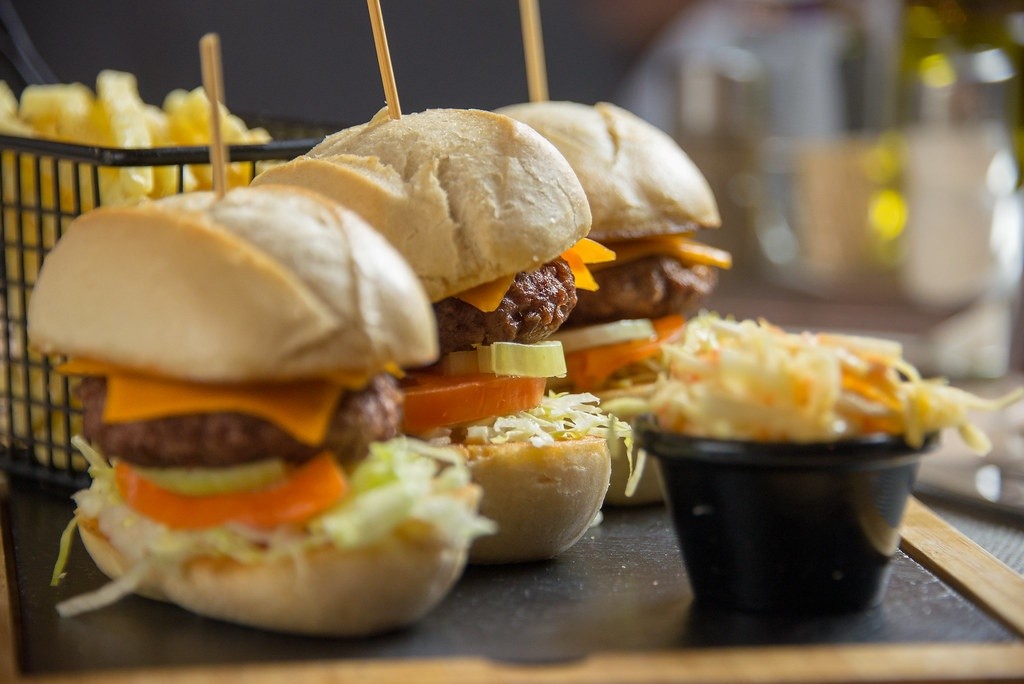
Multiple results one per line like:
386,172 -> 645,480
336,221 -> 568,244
0,125 -> 353,506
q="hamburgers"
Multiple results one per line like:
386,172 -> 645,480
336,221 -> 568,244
28,88 -> 724,638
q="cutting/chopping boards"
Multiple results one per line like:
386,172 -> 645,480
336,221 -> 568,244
0,472 -> 1022,682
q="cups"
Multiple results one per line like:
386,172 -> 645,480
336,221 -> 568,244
634,412 -> 944,617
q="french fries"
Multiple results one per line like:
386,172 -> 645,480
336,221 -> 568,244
1,77 -> 275,469
